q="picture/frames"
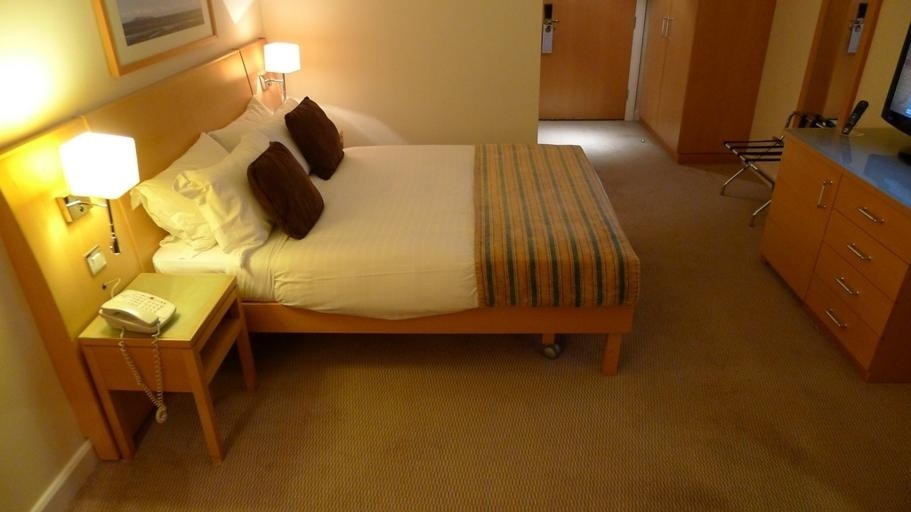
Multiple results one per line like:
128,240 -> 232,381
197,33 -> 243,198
92,0 -> 219,79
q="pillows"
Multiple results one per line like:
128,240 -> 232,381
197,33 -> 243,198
128,132 -> 231,251
208,93 -> 274,152
283,95 -> 345,179
261,96 -> 311,175
173,126 -> 275,253
247,140 -> 324,239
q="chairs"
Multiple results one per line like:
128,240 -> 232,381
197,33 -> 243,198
720,110 -> 838,228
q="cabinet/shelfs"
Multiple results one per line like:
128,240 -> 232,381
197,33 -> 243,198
757,122 -> 911,386
636,0 -> 778,166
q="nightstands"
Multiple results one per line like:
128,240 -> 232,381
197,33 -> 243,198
73,272 -> 257,463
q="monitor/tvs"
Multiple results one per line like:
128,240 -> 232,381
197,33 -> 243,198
881,18 -> 911,169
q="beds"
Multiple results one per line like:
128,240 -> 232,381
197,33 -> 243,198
81,48 -> 643,380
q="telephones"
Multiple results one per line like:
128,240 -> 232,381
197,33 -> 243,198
98,289 -> 177,334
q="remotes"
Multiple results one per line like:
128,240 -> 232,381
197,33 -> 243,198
842,100 -> 868,134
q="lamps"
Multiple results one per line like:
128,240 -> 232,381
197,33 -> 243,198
54,128 -> 139,256
257,41 -> 303,102
